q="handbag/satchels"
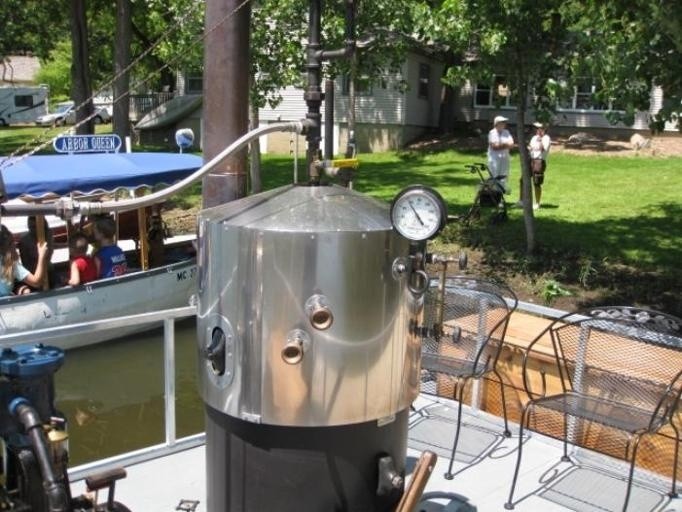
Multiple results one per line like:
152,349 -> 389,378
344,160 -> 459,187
532,159 -> 543,173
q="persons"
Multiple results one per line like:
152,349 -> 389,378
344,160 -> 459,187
510,120 -> 551,209
17,213 -> 61,291
1,223 -> 49,296
487,116 -> 515,194
90,214 -> 129,280
55,234 -> 99,287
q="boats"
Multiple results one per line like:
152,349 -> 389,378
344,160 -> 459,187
0,264 -> 682,512
1,127 -> 205,354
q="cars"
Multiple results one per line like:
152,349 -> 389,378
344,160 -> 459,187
35,103 -> 110,126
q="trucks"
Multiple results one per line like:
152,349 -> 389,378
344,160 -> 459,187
0,84 -> 49,129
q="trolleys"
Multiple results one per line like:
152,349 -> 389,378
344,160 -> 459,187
462,161 -> 510,229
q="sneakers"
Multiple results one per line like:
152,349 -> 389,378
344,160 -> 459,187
533,203 -> 541,210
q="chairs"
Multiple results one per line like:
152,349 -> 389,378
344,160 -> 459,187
416,275 -> 517,479
504,306 -> 682,512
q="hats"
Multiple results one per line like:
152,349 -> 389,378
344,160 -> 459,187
533,121 -> 543,127
493,115 -> 509,126
